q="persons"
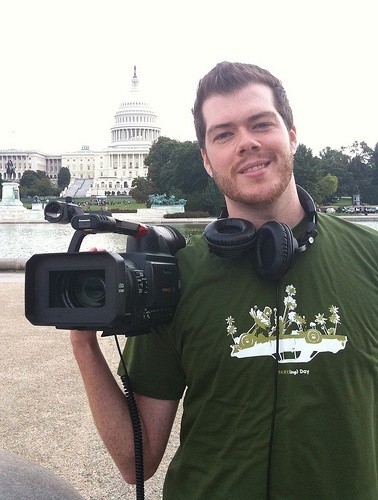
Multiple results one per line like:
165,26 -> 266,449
70,61 -> 377,500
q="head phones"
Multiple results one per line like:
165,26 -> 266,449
204,184 -> 320,284
